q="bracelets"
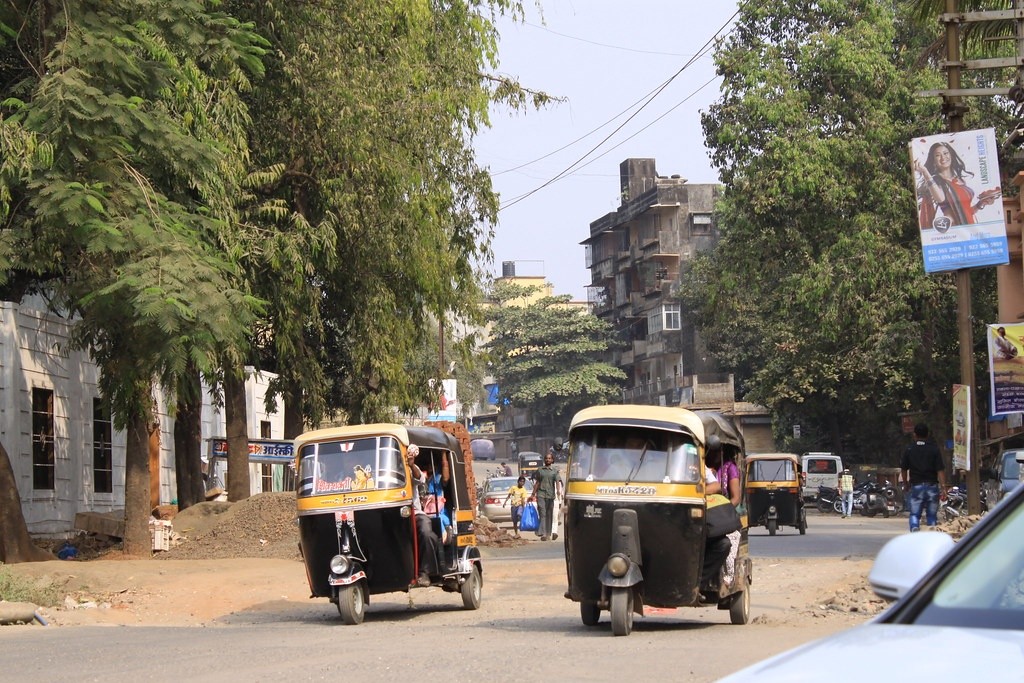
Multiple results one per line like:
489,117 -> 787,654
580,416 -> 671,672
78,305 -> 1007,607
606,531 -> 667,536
977,201 -> 985,210
925,177 -> 936,187
532,493 -> 535,495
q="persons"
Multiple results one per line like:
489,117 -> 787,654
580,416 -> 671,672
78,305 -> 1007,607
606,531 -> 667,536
530,452 -> 564,540
994,327 -> 1018,360
900,423 -> 947,532
913,141 -> 995,229
838,469 -> 855,518
500,462 -> 512,477
371,445 -> 452,586
502,476 -> 527,538
600,434 -> 741,602
428,380 -> 457,412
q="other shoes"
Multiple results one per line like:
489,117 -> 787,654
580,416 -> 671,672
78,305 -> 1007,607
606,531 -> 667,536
417,577 -> 430,586
841,513 -> 846,520
910,524 -> 918,532
724,573 -> 734,588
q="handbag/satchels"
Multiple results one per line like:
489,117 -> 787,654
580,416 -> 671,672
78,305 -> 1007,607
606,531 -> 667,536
520,500 -> 539,531
702,493 -> 742,538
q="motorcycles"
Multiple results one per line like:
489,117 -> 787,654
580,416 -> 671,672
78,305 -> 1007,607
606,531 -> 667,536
518,451 -> 543,482
292,423 -> 484,627
561,405 -> 753,637
942,484 -> 987,524
475,466 -> 508,502
745,453 -> 808,536
816,472 -> 906,518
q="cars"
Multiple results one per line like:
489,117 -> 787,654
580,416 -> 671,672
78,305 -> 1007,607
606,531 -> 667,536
713,480 -> 1024,683
478,476 -> 538,524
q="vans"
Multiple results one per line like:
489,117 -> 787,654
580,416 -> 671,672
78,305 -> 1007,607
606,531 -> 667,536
989,449 -> 1021,503
801,451 -> 844,499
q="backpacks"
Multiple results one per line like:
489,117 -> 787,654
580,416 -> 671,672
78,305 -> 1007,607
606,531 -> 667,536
842,475 -> 853,494
